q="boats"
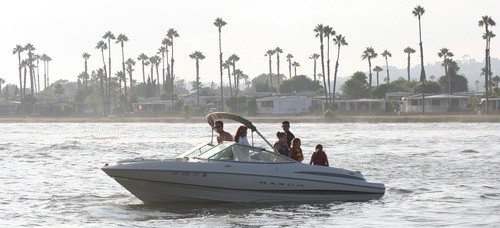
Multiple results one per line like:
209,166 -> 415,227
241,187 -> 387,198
100,140 -> 387,207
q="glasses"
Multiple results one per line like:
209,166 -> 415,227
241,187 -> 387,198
214,125 -> 220,128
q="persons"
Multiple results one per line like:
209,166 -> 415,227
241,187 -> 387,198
214,120 -> 233,159
310,144 -> 329,167
274,120 -> 304,162
235,126 -> 250,161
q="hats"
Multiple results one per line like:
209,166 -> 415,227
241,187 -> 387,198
282,121 -> 290,127
